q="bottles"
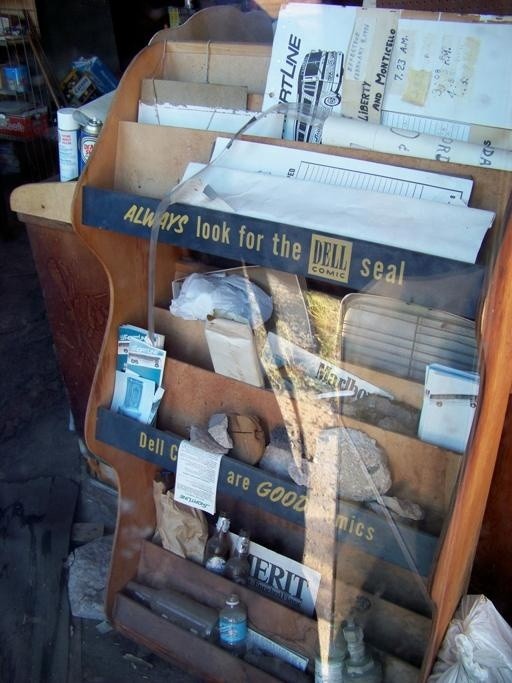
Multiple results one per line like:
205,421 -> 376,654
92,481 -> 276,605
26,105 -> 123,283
202,510 -> 229,575
224,527 -> 251,585
219,595 -> 248,657
72,110 -> 103,179
125,580 -> 222,644
57,108 -> 79,182
